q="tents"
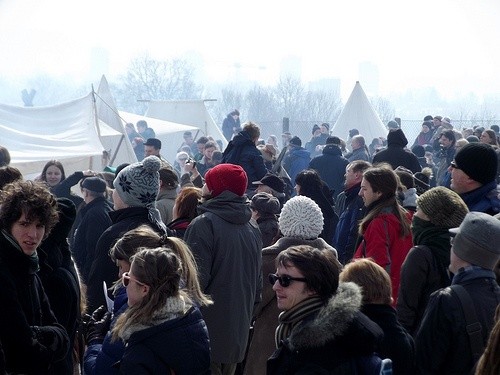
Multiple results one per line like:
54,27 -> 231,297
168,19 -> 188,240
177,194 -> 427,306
0,73 -> 228,181
329,81 -> 389,147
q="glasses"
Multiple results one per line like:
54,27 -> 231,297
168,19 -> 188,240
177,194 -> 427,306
123,272 -> 145,286
450,161 -> 459,169
269,274 -> 307,287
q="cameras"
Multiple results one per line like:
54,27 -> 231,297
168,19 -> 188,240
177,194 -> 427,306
185,157 -> 196,169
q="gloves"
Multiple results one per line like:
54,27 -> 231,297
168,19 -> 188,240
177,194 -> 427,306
85,305 -> 113,345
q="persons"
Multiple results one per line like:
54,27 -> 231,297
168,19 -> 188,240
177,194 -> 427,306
222,109 -> 242,142
0,116 -> 500,375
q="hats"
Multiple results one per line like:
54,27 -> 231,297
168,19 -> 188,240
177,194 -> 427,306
143,138 -> 161,146
387,120 -> 408,147
321,123 -> 329,130
81,177 -> 107,192
49,197 -> 77,238
394,165 -> 416,190
251,192 -> 281,214
435,116 -> 442,121
454,142 -> 496,183
205,163 -> 248,196
312,124 -> 320,135
113,155 -> 161,207
448,212 -> 500,270
422,122 -> 432,129
279,195 -> 324,240
413,167 -> 433,189
415,185 -> 470,230
251,173 -> 285,192
